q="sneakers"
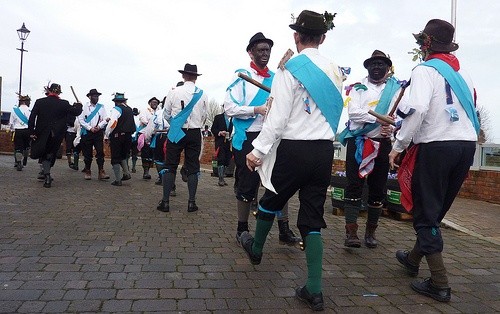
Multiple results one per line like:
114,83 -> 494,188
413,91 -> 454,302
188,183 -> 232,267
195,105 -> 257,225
279,229 -> 302,246
235,232 -> 242,248
410,278 -> 451,302
296,284 -> 325,311
240,232 -> 263,265
396,250 -> 418,276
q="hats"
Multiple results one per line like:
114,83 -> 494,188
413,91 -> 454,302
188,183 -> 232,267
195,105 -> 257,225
246,32 -> 273,52
289,10 -> 326,35
413,19 -> 459,52
112,92 -> 127,102
148,96 -> 167,104
178,63 -> 203,76
363,50 -> 392,70
86,89 -> 102,98
46,84 -> 62,93
132,108 -> 140,115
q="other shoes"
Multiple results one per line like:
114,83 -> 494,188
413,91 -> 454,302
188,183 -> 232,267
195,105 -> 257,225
169,190 -> 176,196
218,180 -> 228,186
112,173 -> 131,186
142,174 -> 162,185
37,172 -> 54,188
180,167 -> 187,182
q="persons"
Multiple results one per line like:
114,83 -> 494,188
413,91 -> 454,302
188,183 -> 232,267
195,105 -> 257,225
211,104 -> 234,185
337,49 -> 406,248
388,19 -> 480,303
27,83 -> 73,187
224,32 -> 305,247
125,97 -> 162,178
239,11 -> 345,310
9,95 -> 36,171
154,64 -> 209,212
78,89 -> 110,180
104,92 -> 138,185
66,118 -> 81,170
202,125 -> 212,138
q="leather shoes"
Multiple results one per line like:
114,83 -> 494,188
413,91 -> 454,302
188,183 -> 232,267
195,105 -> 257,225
188,201 -> 198,212
157,200 -> 169,212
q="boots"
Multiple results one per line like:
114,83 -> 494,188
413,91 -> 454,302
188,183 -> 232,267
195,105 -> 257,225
84,167 -> 110,179
67,155 -> 79,170
14,155 -> 27,171
345,223 -> 379,248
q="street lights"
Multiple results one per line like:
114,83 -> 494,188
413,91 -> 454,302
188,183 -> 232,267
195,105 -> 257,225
16,22 -> 30,105
